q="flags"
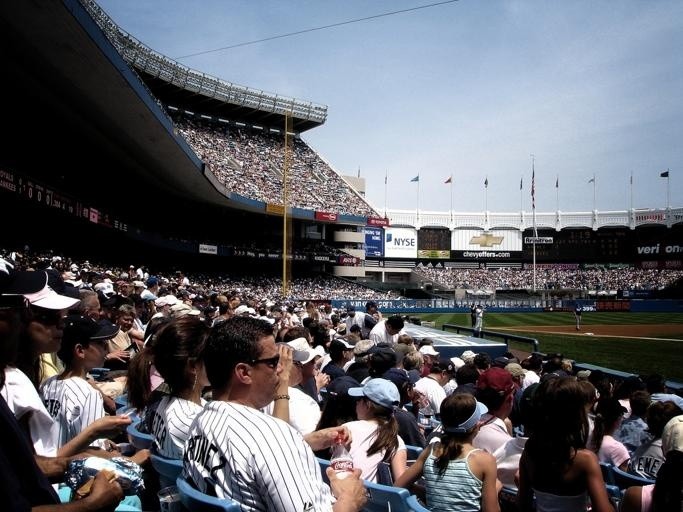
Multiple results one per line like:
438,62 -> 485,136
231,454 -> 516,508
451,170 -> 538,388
532,169 -> 535,208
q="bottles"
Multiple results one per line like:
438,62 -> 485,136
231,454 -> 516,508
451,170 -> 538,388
329,432 -> 353,479
413,389 -> 430,408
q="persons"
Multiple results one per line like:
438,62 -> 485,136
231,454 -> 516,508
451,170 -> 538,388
1,243 -> 683,512
172,114 -> 379,220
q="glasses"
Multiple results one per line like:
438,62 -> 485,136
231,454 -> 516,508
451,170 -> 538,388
249,354 -> 279,368
